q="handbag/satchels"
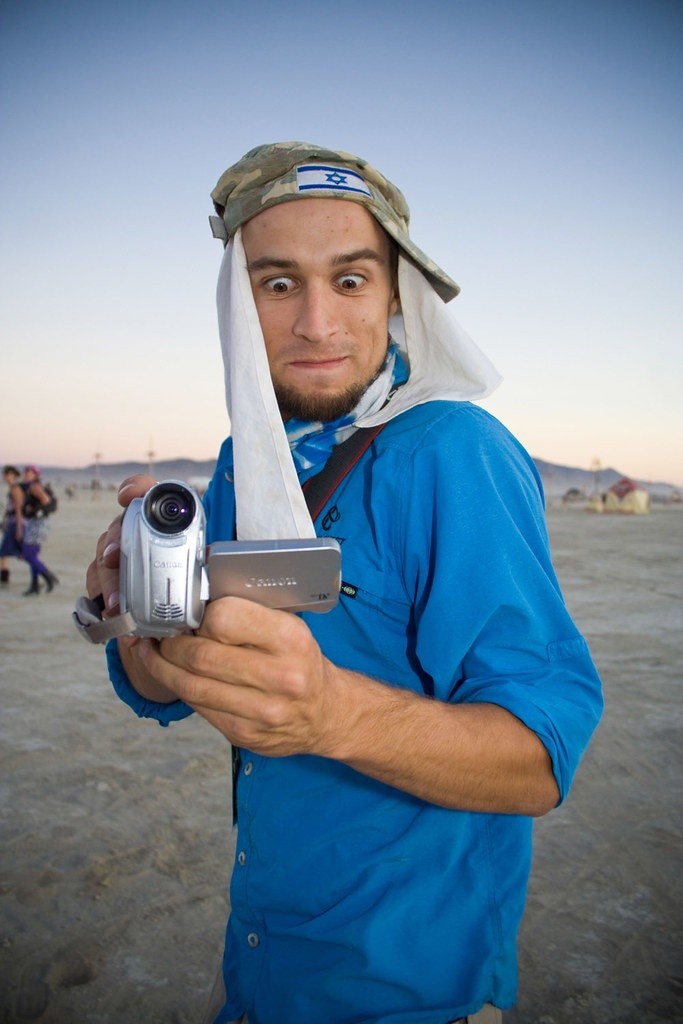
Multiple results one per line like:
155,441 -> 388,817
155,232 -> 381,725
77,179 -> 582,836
31,484 -> 58,512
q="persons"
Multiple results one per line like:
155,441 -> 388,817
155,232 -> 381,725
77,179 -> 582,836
0,466 -> 25,585
19,465 -> 62,596
86,143 -> 606,1024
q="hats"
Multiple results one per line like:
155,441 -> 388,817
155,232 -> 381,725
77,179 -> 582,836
209,142 -> 459,304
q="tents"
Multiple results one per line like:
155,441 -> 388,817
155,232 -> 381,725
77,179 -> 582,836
587,478 -> 648,514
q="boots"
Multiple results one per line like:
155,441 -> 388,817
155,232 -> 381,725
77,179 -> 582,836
25,579 -> 40,595
43,569 -> 59,592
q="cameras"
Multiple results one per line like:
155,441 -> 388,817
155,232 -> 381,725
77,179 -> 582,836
119,478 -> 342,641
